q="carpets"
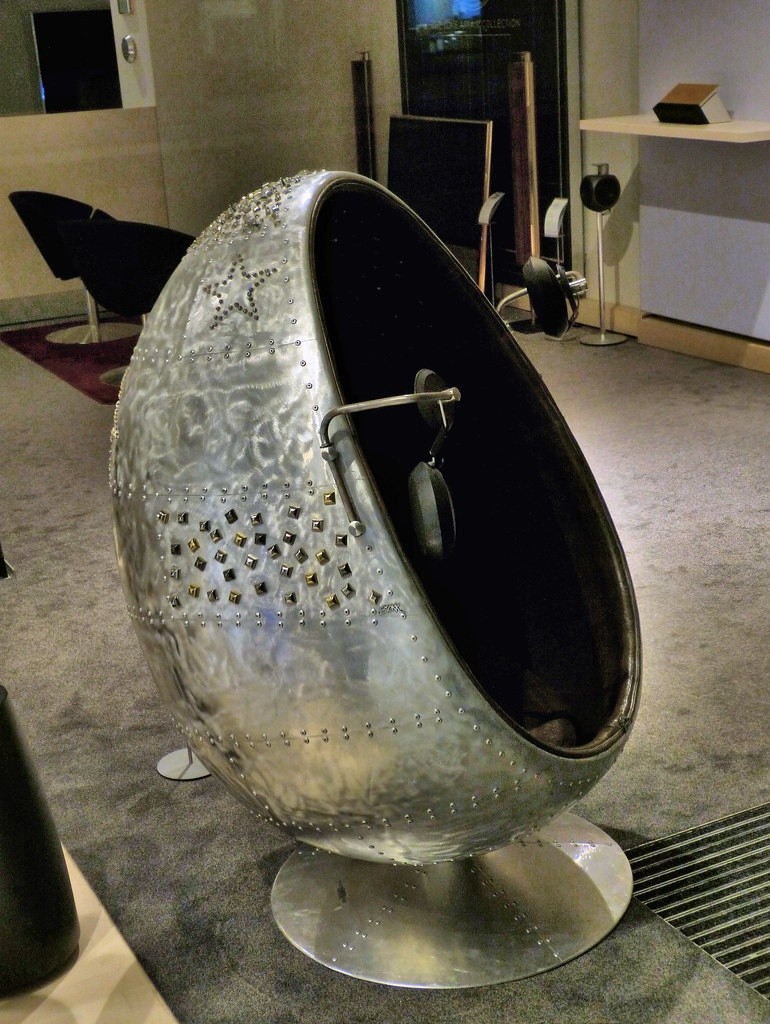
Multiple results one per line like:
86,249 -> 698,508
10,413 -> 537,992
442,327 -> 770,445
0,315 -> 144,406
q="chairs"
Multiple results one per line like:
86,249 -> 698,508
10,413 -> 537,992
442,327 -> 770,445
8,191 -> 197,389
